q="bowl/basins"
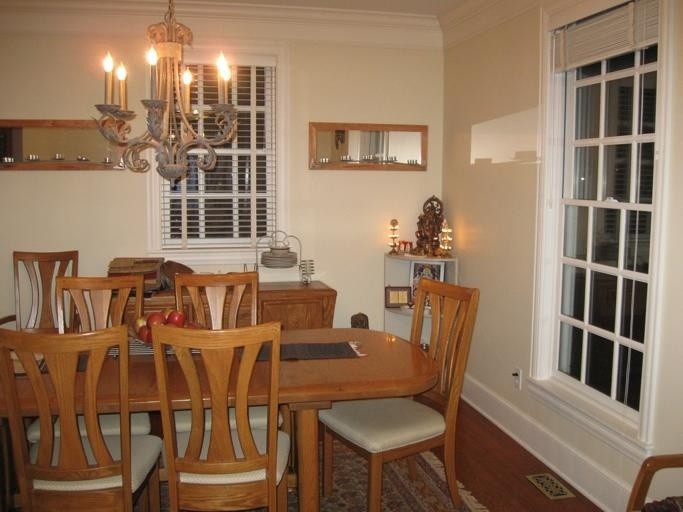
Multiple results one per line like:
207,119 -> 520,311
129,330 -> 171,351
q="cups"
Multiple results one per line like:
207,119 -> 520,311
319,155 -> 417,166
1,152 -> 112,164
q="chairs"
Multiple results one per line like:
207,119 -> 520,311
626,452 -> 683,512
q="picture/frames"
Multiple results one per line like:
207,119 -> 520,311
332,130 -> 349,156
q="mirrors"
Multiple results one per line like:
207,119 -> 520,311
309,121 -> 429,171
0,119 -> 128,171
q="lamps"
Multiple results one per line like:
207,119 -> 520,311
87,1 -> 240,189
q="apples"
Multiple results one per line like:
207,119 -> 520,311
135,307 -> 197,345
